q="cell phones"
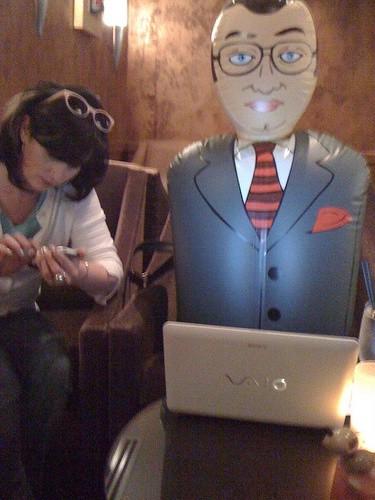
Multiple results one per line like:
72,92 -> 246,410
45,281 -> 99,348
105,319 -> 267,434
28,245 -> 77,270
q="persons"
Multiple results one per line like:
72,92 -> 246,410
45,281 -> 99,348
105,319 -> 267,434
1,80 -> 124,500
168,0 -> 369,336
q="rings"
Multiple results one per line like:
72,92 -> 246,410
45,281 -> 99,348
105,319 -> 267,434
62,271 -> 66,277
55,274 -> 63,281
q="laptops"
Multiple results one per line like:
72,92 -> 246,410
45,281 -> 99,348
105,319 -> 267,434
162,320 -> 359,429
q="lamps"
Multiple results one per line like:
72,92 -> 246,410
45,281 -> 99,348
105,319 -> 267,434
102,0 -> 127,70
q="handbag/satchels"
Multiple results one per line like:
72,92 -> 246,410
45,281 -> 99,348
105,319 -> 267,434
0,263 -> 41,318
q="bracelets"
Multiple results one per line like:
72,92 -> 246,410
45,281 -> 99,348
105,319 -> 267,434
80,261 -> 87,286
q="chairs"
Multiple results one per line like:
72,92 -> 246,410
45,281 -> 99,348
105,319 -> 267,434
35,157 -> 179,500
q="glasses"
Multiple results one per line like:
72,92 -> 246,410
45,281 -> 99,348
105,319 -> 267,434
39,88 -> 115,133
